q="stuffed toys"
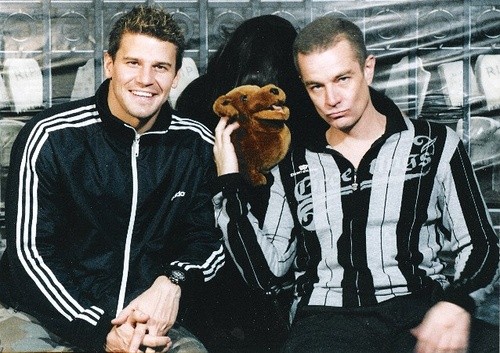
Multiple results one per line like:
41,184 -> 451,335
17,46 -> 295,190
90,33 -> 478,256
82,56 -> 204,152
212,83 -> 292,188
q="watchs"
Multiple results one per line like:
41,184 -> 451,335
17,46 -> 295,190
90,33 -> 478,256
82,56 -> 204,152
156,268 -> 188,295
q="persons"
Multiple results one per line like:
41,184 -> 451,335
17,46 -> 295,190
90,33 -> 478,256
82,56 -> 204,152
1,9 -> 230,352
206,16 -> 500,353
